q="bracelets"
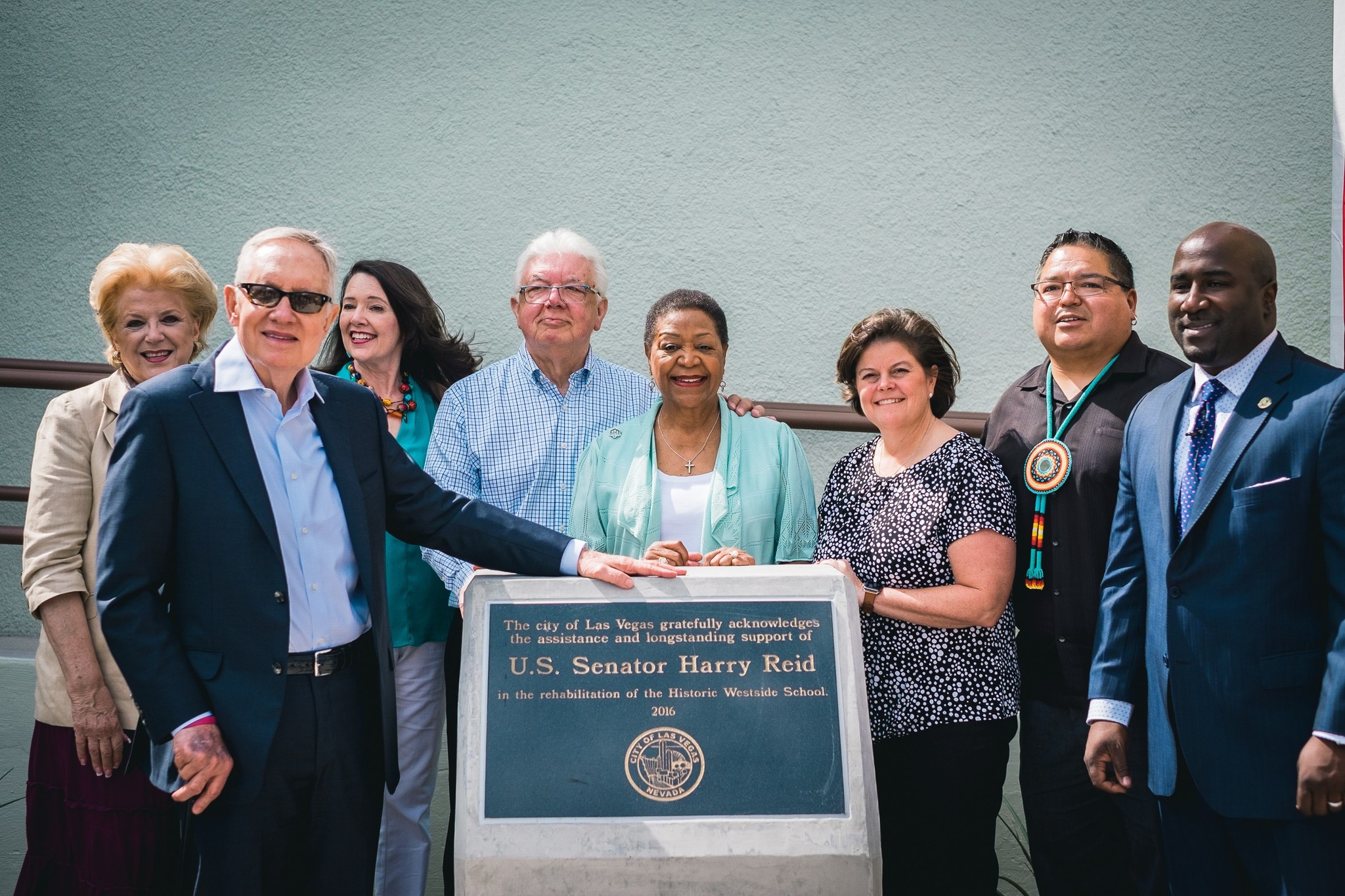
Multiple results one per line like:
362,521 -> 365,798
183,716 -> 216,729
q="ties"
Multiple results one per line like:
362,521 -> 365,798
1175,378 -> 1229,544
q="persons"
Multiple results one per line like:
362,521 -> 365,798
1082,221 -> 1344,896
418,226 -> 762,896
11,242 -> 221,896
566,287 -> 819,567
812,305 -> 1024,896
308,258 -> 488,896
979,227 -> 1172,896
93,225 -> 687,896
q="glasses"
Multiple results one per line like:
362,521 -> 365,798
519,284 -> 601,305
237,283 -> 332,314
1031,275 -> 1133,301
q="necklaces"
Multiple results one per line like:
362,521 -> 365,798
658,404 -> 720,474
347,361 -> 418,424
1025,354 -> 1121,591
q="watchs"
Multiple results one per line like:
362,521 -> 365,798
861,580 -> 883,614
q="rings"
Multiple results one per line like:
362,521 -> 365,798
1328,801 -> 1342,808
658,556 -> 668,564
728,550 -> 739,558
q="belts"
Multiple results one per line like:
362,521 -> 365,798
287,630 -> 371,677
